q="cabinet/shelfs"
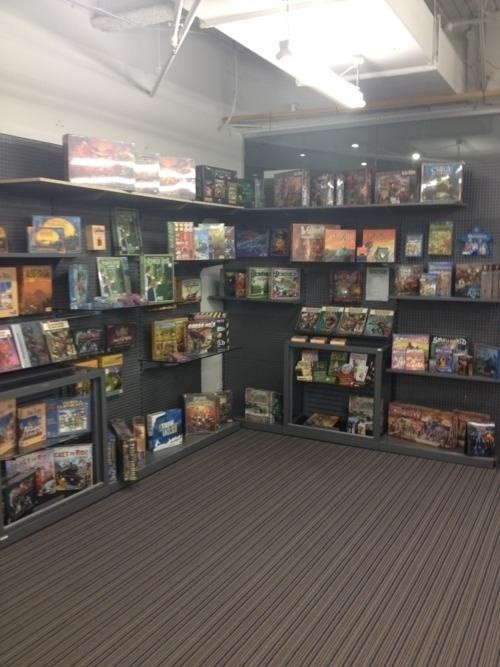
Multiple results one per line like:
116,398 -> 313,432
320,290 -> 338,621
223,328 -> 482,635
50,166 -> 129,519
1,177 -> 498,541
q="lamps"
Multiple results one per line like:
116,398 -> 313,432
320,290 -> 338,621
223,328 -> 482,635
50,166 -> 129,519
181,0 -> 366,111
